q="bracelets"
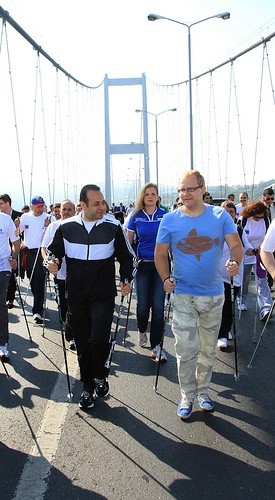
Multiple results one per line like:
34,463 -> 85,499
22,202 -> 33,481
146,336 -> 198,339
163,277 -> 170,284
230,259 -> 240,266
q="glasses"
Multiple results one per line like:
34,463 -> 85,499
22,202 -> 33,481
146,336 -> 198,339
253,215 -> 264,219
177,184 -> 203,193
264,197 -> 274,200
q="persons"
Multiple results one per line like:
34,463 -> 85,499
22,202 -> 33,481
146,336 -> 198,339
0,171 -> 275,419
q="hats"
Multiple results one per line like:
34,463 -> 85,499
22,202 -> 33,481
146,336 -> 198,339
32,196 -> 45,206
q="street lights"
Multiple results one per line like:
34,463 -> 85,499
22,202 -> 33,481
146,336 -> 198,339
147,11 -> 231,168
135,108 -> 178,187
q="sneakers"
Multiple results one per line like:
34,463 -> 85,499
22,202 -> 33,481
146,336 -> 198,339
0,346 -> 9,358
218,338 -> 227,347
138,329 -> 147,346
260,311 -> 272,320
177,399 -> 194,417
196,393 -> 214,410
79,390 -> 95,408
153,347 -> 167,361
95,376 -> 109,397
236,296 -> 247,311
228,332 -> 233,339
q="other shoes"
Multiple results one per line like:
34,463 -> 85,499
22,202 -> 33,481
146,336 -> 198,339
7,301 -> 13,309
69,339 -> 76,349
34,314 -> 42,324
